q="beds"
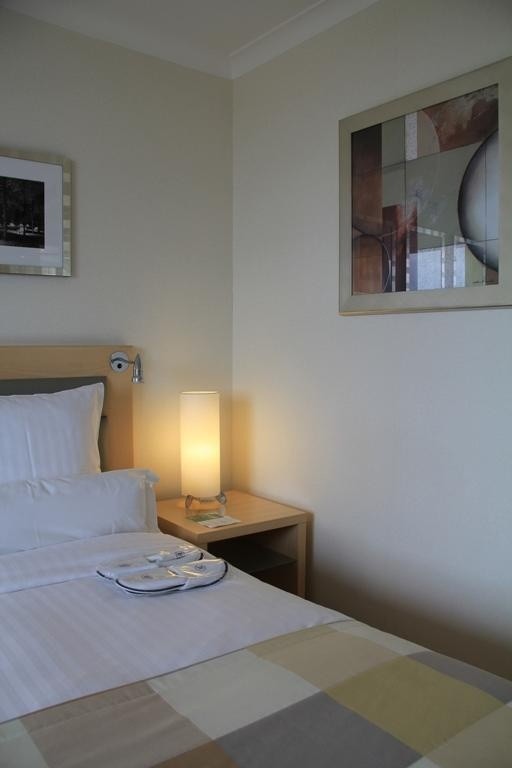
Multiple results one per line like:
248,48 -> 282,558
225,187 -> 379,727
0,344 -> 512,768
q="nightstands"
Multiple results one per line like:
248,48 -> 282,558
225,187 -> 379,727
155,488 -> 310,602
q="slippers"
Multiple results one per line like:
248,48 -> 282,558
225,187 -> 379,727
98,546 -> 228,597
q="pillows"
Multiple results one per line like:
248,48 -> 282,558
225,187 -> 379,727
0,380 -> 107,484
0,468 -> 160,556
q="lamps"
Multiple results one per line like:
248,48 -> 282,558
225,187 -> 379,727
177,391 -> 228,509
110,350 -> 144,385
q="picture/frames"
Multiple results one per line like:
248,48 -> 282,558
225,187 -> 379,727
0,144 -> 76,277
339,56 -> 512,315
